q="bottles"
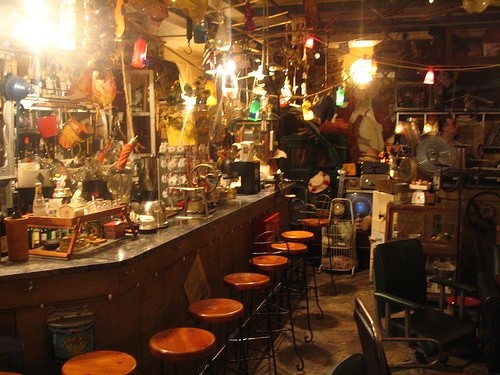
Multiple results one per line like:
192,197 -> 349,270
32,183 -> 46,216
193,17 -> 208,44
52,181 -> 72,204
26,227 -> 72,249
45,63 -> 68,96
131,34 -> 148,68
279,93 -> 288,109
335,86 -> 345,106
301,98 -> 314,121
313,94 -> 319,119
247,97 -> 260,122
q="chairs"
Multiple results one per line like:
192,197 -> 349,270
329,237 -> 500,375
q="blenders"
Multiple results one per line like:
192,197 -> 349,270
134,156 -> 165,219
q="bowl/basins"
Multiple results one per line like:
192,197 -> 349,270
432,262 -> 456,280
406,233 -> 419,239
41,240 -> 60,250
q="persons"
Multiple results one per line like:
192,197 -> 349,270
437,116 -> 474,166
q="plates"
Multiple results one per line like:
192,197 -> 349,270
422,239 -> 449,244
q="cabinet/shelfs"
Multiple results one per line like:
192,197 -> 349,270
394,111 -> 500,187
386,203 -> 457,301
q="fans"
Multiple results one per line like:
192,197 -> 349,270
415,132 -> 455,191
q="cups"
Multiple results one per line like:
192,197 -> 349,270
392,230 -> 399,239
87,198 -> 111,214
38,115 -> 58,138
138,215 -> 157,234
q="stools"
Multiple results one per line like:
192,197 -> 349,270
0,218 -> 335,375
